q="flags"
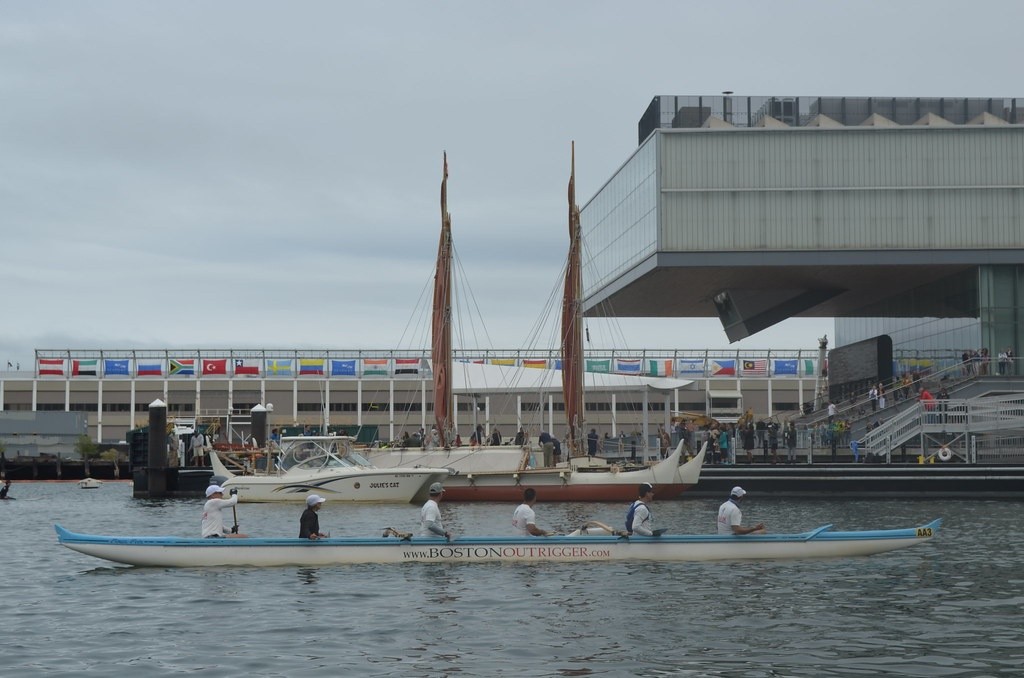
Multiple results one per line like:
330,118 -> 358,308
331,360 -> 356,377
773,359 -> 930,376
679,360 -> 705,376
394,359 -> 419,374
299,358 -> 324,375
202,359 -> 227,375
266,360 -> 292,376
363,359 -> 388,376
39,359 -> 64,376
169,360 -> 195,375
104,360 -> 130,376
72,360 -> 97,377
461,358 -> 673,377
711,360 -> 736,375
742,360 -> 768,375
137,360 -> 163,377
235,359 -> 260,375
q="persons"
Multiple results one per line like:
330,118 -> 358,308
168,370 -> 949,468
511,488 -> 548,536
201,485 -> 249,539
625,482 -> 668,536
961,347 -> 1014,375
420,482 -> 452,542
299,495 -> 328,540
717,486 -> 764,535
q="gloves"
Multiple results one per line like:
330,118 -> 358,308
230,489 -> 238,496
231,524 -> 239,534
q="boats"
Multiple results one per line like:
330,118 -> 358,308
52,516 -> 945,567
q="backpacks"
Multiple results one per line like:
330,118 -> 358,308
625,501 -> 650,531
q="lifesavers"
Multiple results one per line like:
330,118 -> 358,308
938,447 -> 951,461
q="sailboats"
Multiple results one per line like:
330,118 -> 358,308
209,141 -> 708,505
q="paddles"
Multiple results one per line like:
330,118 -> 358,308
653,528 -> 669,533
231,494 -> 238,534
317,531 -> 331,539
384,526 -> 411,542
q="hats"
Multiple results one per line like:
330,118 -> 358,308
730,486 -> 747,497
639,482 -> 654,497
205,485 -> 225,498
429,482 -> 445,493
790,423 -> 794,426
306,494 -> 326,506
